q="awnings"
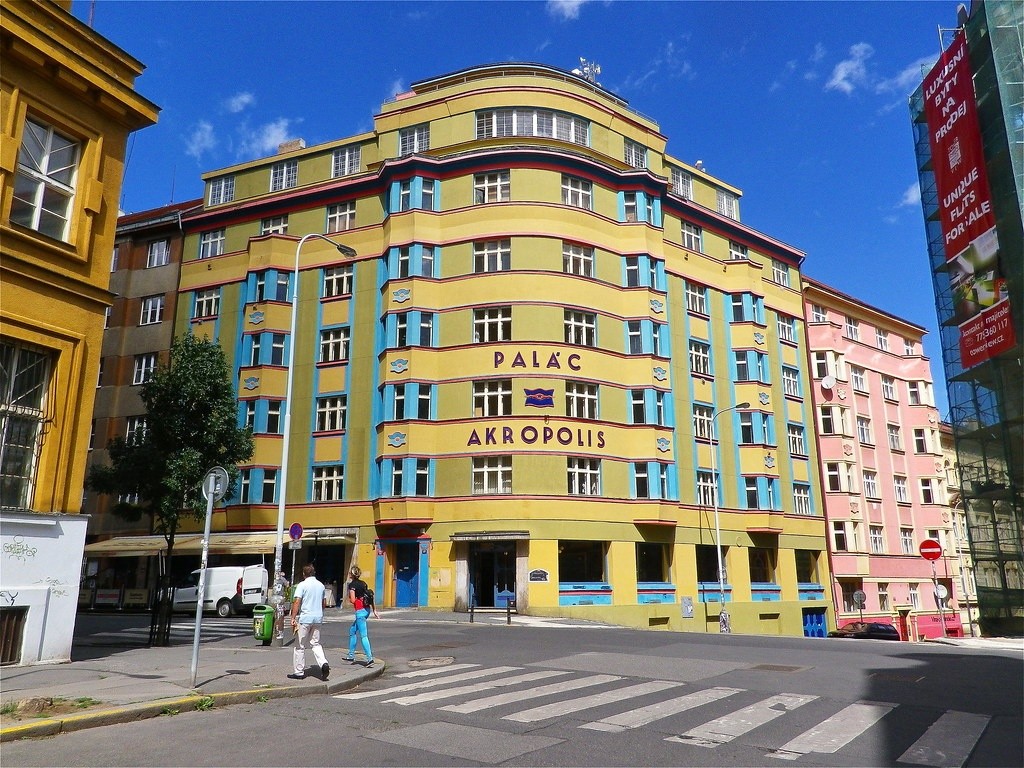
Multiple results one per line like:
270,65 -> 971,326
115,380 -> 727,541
163,534 -> 311,616
80,535 -> 205,576
450,530 -> 531,542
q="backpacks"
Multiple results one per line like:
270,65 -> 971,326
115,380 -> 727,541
356,581 -> 374,606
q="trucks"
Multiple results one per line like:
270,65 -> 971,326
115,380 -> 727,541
157,564 -> 268,618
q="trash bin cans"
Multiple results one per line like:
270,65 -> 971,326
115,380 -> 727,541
252,604 -> 275,640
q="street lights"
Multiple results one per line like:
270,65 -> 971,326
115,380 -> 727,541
710,402 -> 750,633
272,234 -> 358,647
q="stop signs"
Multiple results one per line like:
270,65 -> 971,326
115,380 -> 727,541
919,540 -> 942,561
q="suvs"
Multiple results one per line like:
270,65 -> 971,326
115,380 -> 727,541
827,622 -> 900,641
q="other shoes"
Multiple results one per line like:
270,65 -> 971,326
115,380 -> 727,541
364,660 -> 374,667
287,674 -> 304,679
342,657 -> 354,663
322,663 -> 329,679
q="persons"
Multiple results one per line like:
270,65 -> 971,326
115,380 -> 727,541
286,564 -> 332,680
341,566 -> 380,668
279,572 -> 289,595
